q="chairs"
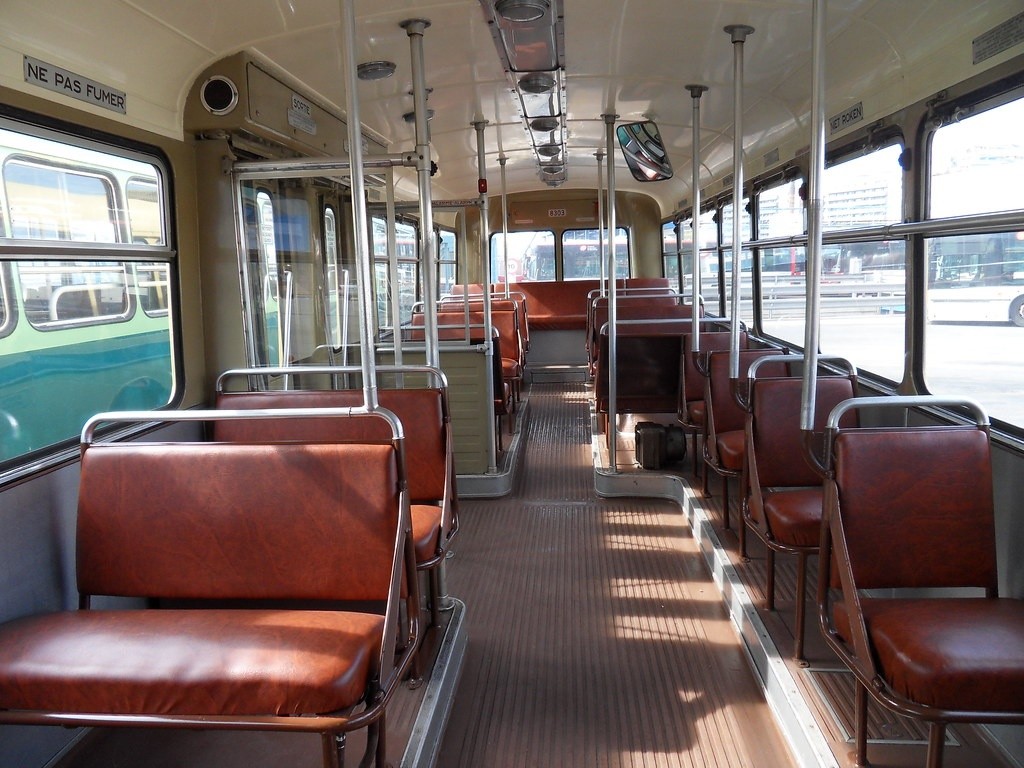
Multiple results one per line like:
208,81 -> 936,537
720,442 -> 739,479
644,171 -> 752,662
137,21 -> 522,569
682,330 -> 1024,768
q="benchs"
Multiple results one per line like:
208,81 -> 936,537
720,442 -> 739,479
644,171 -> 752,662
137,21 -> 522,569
0,278 -> 747,768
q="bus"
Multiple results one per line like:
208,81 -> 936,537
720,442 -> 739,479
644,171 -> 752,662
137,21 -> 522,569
922,230 -> 1024,327
518,237 -> 708,303
1,124 -> 381,464
